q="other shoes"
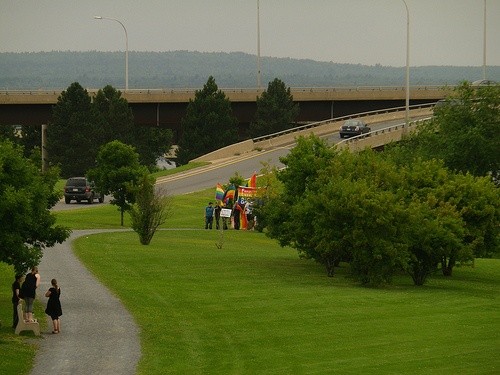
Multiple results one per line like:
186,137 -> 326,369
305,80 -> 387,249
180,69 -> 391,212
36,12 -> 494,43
52,330 -> 58,334
58,329 -> 61,333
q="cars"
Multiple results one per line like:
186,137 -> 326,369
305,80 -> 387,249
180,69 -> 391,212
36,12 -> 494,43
340,119 -> 371,138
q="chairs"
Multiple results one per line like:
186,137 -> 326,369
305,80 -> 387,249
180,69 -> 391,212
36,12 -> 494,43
15,303 -> 40,336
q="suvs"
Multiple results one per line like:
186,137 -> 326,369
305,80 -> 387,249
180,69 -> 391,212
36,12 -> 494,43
64,177 -> 104,204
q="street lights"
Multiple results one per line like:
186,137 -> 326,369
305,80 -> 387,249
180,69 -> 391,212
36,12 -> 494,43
93,16 -> 128,89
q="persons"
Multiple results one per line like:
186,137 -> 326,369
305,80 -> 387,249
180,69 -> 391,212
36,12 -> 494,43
45,279 -> 63,334
24,266 -> 40,323
219,202 -> 228,230
232,198 -> 258,230
12,272 -> 23,329
204,202 -> 214,230
213,201 -> 221,230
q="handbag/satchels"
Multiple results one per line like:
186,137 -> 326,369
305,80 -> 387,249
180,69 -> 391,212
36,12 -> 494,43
18,289 -> 25,299
